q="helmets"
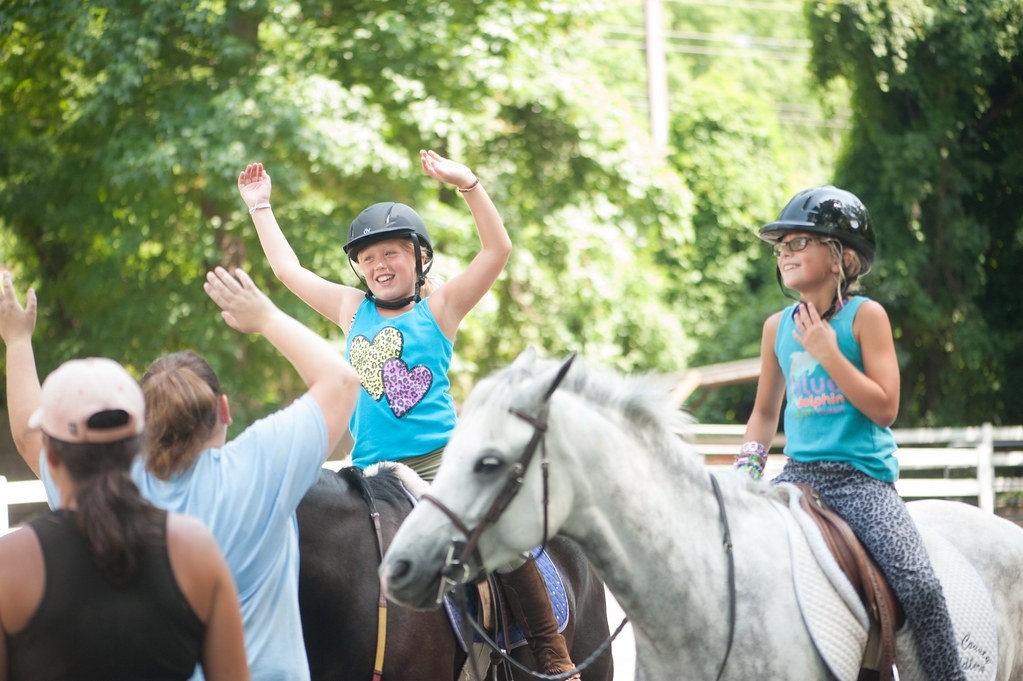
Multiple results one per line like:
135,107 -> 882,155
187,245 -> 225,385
344,202 -> 433,264
757,183 -> 876,278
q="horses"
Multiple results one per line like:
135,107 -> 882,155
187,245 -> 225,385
297,466 -> 617,680
374,346 -> 1023,681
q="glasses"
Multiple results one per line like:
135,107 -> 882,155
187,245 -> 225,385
772,236 -> 826,259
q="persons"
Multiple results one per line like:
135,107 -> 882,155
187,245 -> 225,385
0,356 -> 251,681
733,183 -> 967,681
0,265 -> 360,681
237,149 -> 581,681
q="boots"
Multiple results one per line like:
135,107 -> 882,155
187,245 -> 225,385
496,552 -> 582,681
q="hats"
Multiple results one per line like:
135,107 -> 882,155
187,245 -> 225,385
27,355 -> 149,444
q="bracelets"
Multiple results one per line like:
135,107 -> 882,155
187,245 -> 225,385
733,441 -> 768,479
248,203 -> 272,213
458,177 -> 480,193
